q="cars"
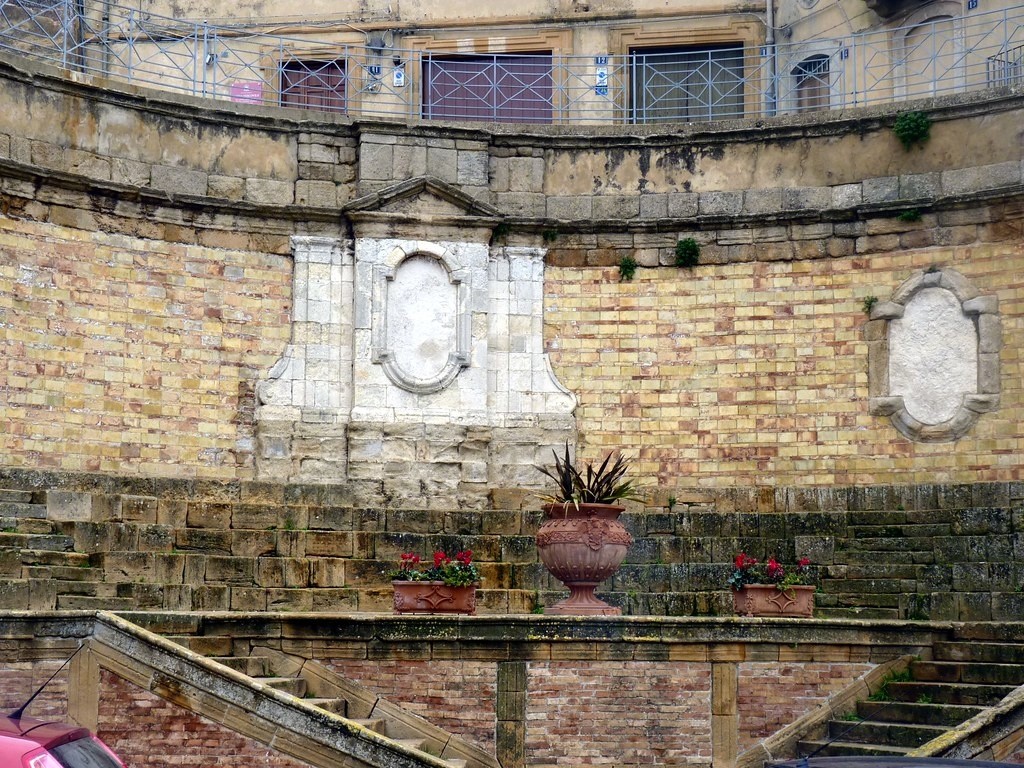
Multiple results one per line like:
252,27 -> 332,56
0,712 -> 130,768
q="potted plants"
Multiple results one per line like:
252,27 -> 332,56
537,439 -> 635,615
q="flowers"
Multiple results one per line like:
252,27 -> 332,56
386,549 -> 484,587
725,553 -> 811,588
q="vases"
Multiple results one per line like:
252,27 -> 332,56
390,580 -> 477,616
729,584 -> 816,618
